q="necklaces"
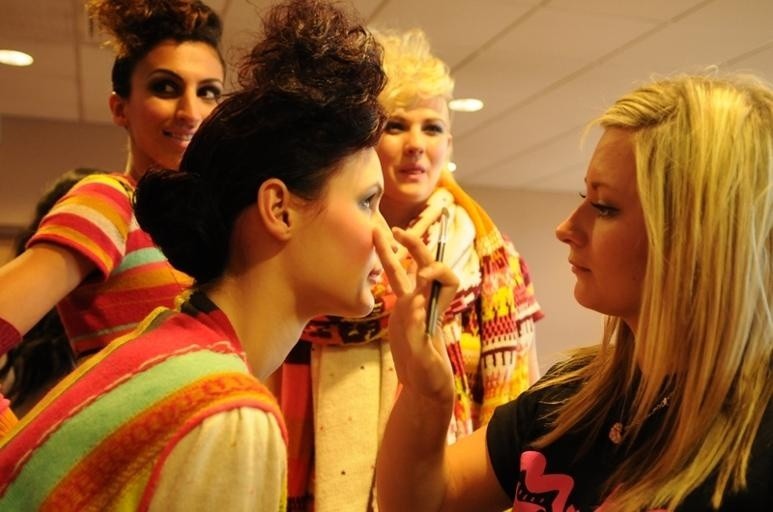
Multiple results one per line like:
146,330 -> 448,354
606,376 -> 676,444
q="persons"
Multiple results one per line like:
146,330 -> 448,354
0,1 -> 397,512
0,1 -> 226,443
367,68 -> 772,511
263,26 -> 543,512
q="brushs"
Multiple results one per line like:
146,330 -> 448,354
425,208 -> 450,336
